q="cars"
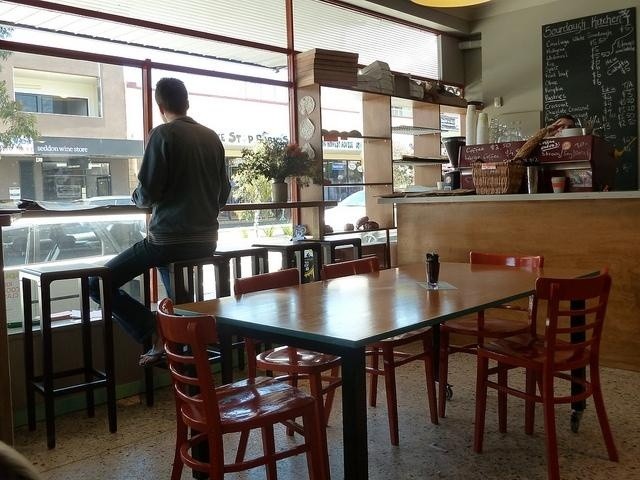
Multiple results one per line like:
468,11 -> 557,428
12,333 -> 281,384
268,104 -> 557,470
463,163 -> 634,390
325,190 -> 397,233
75,195 -> 138,207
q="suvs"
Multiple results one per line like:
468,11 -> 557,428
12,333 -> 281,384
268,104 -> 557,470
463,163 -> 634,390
1,213 -> 167,324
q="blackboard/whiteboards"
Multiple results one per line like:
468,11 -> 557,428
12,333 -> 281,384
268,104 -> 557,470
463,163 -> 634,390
541,6 -> 640,190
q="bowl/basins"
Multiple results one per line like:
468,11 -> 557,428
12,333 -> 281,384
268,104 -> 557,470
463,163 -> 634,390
561,128 -> 582,136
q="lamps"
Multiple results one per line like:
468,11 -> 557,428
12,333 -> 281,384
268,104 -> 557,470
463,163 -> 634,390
411,0 -> 490,8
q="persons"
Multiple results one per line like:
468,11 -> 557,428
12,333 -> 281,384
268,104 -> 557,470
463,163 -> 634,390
88,79 -> 231,367
546,115 -> 579,138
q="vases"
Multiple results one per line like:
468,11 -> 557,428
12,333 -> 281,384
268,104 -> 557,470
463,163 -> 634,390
270,179 -> 288,202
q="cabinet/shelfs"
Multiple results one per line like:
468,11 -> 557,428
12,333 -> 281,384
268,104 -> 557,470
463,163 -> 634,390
295,82 -> 467,271
375,189 -> 640,373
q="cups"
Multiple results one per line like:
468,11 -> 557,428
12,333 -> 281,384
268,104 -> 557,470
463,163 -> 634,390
477,113 -> 489,144
426,263 -> 440,286
490,118 -> 522,143
466,105 -> 476,145
552,177 -> 565,193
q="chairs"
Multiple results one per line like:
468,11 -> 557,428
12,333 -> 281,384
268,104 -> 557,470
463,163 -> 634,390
320,256 -> 439,446
473,265 -> 619,480
437,249 -> 544,433
234,268 -> 342,480
155,297 -> 324,480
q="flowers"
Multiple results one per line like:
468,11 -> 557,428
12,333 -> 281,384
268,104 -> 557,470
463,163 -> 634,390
236,136 -> 324,190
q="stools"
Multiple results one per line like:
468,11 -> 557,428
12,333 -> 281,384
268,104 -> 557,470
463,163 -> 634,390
297,238 -> 362,265
143,256 -> 232,406
251,240 -> 322,356
19,261 -> 118,449
194,247 -> 274,379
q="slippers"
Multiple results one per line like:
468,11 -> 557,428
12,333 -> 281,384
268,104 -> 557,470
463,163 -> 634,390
136,346 -> 189,368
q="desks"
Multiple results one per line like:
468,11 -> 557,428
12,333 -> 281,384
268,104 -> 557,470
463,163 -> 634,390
175,261 -> 601,480
